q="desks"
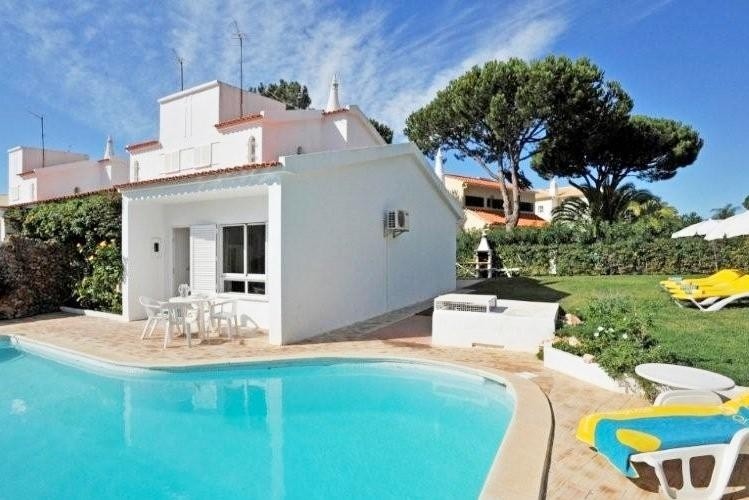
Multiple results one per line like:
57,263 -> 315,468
637,363 -> 738,393
170,295 -> 218,347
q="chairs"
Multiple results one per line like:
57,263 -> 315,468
139,297 -> 240,348
661,268 -> 749,314
560,386 -> 748,499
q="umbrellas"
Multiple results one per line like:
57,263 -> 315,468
703,210 -> 749,243
671,218 -> 726,273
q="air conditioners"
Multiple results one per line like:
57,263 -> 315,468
386,209 -> 411,231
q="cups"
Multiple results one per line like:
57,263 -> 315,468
179,284 -> 190,300
